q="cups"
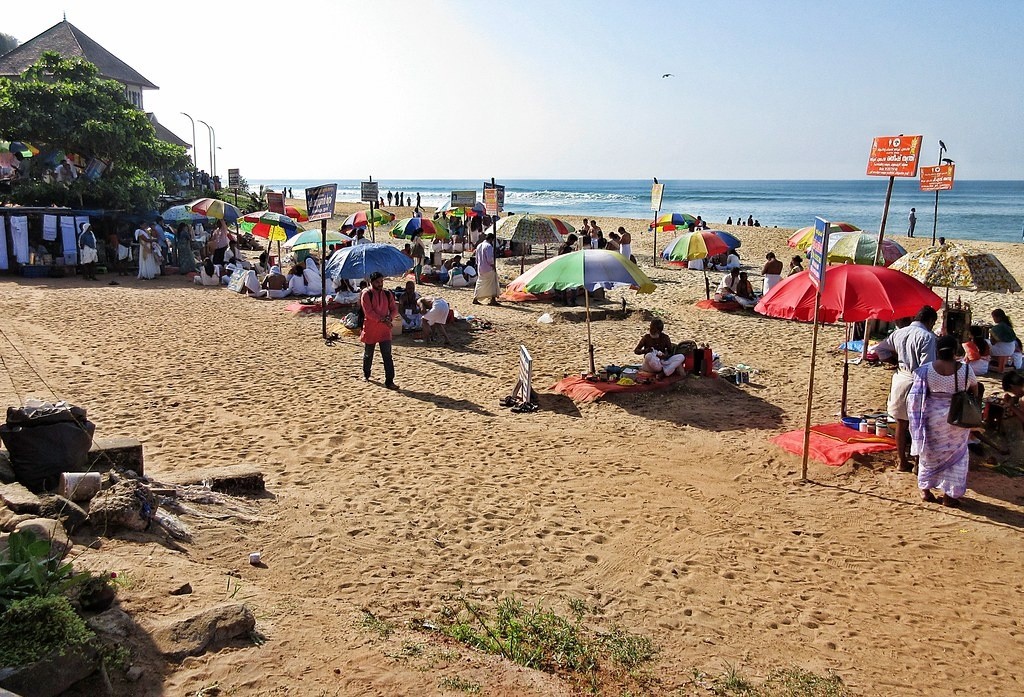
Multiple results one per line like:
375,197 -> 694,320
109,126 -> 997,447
876,424 -> 888,437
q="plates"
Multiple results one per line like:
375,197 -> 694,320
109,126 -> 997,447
617,382 -> 636,386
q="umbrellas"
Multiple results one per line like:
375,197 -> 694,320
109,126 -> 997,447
755,217 -> 1024,418
324,243 -> 416,287
507,248 -> 657,373
648,213 -> 700,238
0,140 -> 40,158
285,204 -> 309,222
661,228 -> 743,300
161,206 -> 215,220
435,198 -> 486,258
390,215 -> 450,240
186,198 -> 243,238
483,211 -> 565,275
235,211 -> 306,273
339,208 -> 396,241
281,228 -> 353,267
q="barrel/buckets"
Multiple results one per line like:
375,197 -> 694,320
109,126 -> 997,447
56,257 -> 64,265
59,471 -> 102,502
29,252 -> 37,265
193,276 -> 200,284
35,255 -> 44,265
448,308 -> 455,321
188,272 -> 196,281
44,254 -> 53,265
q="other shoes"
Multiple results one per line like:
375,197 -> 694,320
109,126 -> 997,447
385,379 -> 398,390
416,279 -> 424,286
364,368 -> 370,380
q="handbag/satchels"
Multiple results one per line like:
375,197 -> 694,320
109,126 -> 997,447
947,364 -> 982,428
645,347 -> 662,370
410,242 -> 424,258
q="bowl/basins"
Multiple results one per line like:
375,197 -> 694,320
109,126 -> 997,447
841,416 -> 862,430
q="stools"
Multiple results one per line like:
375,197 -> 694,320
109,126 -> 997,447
988,355 -> 1015,374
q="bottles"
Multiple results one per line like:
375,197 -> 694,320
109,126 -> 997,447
735,364 -> 750,384
665,347 -> 670,359
600,371 -> 607,381
453,304 -> 459,318
714,353 -> 720,370
671,343 -> 677,356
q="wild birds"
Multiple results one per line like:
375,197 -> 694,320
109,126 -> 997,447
216,147 -> 221,150
939,139 -> 947,153
662,74 -> 675,78
942,158 -> 955,166
653,177 -> 658,185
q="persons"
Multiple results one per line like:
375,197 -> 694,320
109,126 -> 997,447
938,236 -> 946,246
400,208 -> 518,306
11,152 -> 31,179
350,227 -> 366,245
633,252 -> 1024,505
726,214 -> 777,228
148,167 -> 222,195
379,189 -> 425,218
74,216 -> 338,300
355,271 -> 449,391
559,214 -> 633,305
906,207 -> 918,238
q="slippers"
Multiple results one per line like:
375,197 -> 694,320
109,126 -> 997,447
897,461 -> 914,473
510,400 -> 539,413
942,497 -> 960,508
499,395 -> 518,406
109,281 -> 119,285
325,338 -> 332,346
487,300 -> 502,306
919,490 -> 932,502
331,332 -> 341,340
472,300 -> 481,305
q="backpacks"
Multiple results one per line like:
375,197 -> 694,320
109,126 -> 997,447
358,291 -> 390,329
